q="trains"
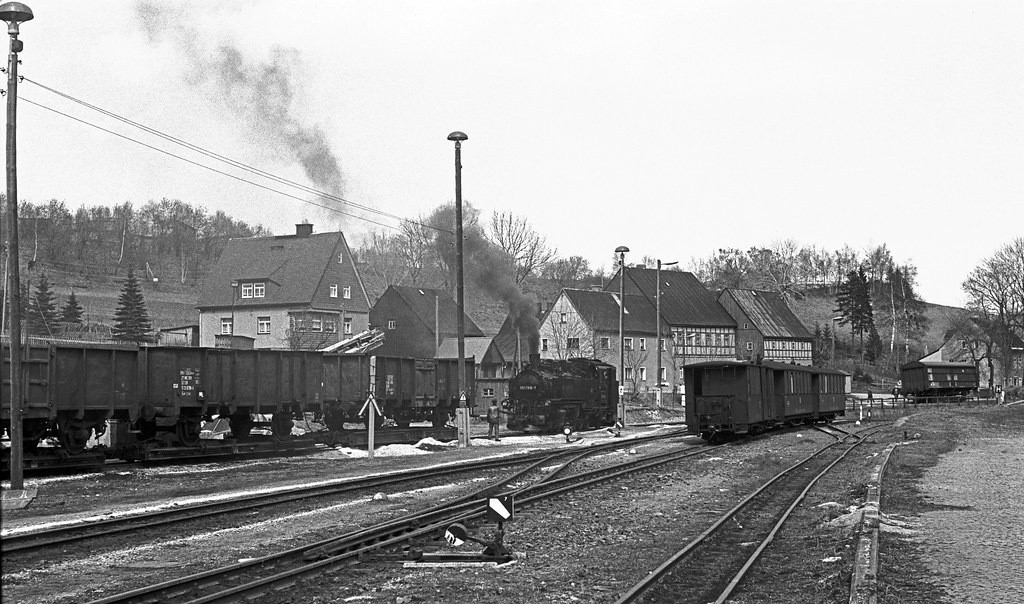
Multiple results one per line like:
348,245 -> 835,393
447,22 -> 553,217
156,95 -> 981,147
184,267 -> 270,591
680,354 -> 846,445
900,361 -> 980,402
0,342 -> 480,456
500,357 -> 619,433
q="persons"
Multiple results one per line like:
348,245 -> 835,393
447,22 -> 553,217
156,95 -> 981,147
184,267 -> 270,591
891,386 -> 900,402
867,388 -> 875,403
607,418 -> 624,437
487,398 -> 512,441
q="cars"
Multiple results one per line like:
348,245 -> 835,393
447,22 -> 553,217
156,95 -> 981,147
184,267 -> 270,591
977,388 -> 995,398
892,387 -> 902,395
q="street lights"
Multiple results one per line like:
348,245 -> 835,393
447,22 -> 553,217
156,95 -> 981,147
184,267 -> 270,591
0,1 -> 34,489
26,260 -> 36,336
832,317 -> 844,368
418,289 -> 439,357
615,246 -> 630,429
230,281 -> 239,335
682,333 -> 696,365
447,131 -> 470,449
656,259 -> 678,408
